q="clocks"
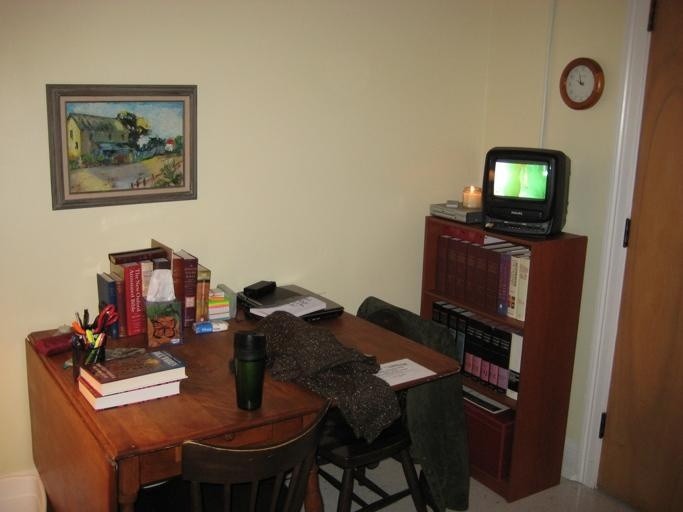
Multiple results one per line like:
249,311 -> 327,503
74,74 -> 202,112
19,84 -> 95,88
559,58 -> 604,111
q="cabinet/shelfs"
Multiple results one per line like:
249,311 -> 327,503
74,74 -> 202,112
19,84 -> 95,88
420,218 -> 587,502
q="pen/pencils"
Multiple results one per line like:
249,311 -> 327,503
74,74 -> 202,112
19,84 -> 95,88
72,309 -> 105,378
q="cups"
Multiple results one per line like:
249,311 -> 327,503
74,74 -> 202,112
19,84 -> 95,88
462,186 -> 482,209
232,329 -> 266,411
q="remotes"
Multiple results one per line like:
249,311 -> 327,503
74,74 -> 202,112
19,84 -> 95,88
446,200 -> 458,208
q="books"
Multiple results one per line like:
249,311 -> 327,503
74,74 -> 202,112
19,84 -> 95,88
97,235 -> 209,338
248,293 -> 327,317
76,375 -> 182,412
78,348 -> 190,396
426,221 -> 532,400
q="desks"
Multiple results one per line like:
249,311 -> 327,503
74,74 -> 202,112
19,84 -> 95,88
25,310 -> 460,512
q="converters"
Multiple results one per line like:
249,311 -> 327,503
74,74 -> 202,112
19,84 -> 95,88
244,281 -> 276,299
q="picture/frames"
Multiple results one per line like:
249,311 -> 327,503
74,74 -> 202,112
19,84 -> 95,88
47,85 -> 197,210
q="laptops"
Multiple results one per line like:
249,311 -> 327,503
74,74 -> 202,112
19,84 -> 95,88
237,285 -> 344,324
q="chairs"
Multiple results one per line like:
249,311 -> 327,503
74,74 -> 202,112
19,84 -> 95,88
138,399 -> 332,512
317,298 -> 465,512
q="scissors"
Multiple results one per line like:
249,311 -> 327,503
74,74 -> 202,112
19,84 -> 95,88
95,305 -> 119,333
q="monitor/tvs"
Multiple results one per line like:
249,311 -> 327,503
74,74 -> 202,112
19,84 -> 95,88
481,147 -> 570,238
